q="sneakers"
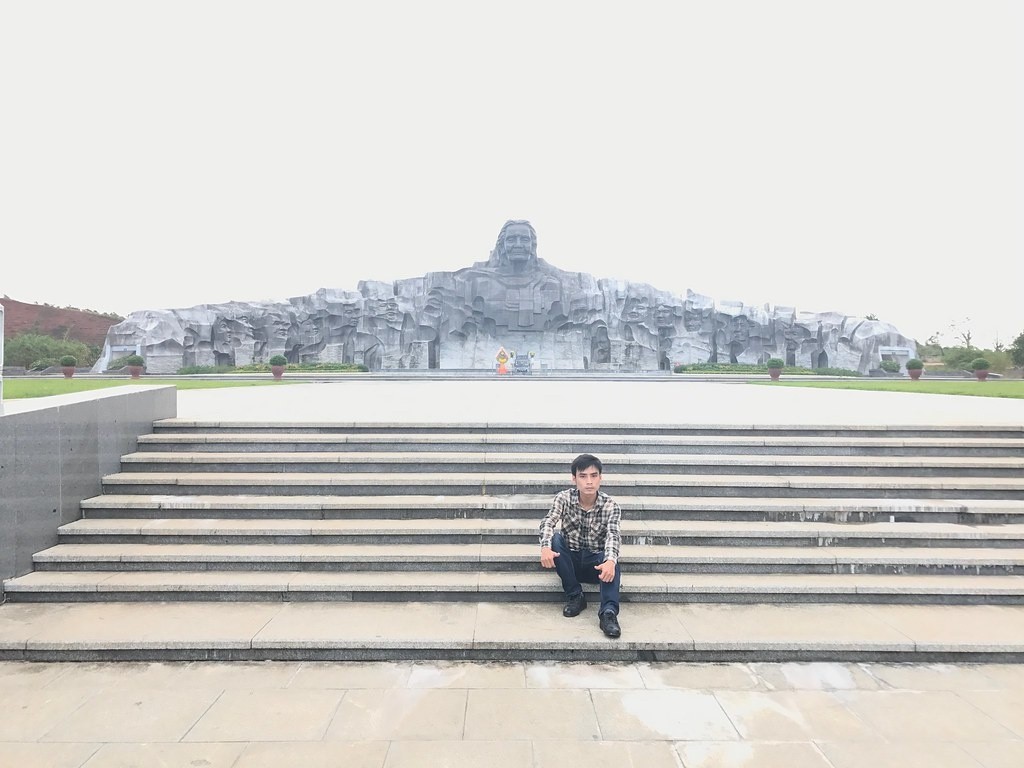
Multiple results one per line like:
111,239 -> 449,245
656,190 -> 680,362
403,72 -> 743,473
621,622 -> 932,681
563,592 -> 587,617
600,612 -> 621,636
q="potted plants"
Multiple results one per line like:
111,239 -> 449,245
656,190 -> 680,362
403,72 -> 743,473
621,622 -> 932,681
767,358 -> 784,379
972,358 -> 992,380
270,355 -> 287,379
126,355 -> 144,378
906,359 -> 924,379
60,355 -> 77,378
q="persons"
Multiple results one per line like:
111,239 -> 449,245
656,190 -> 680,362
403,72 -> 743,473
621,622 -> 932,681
92,219 -> 916,373
539,454 -> 622,637
